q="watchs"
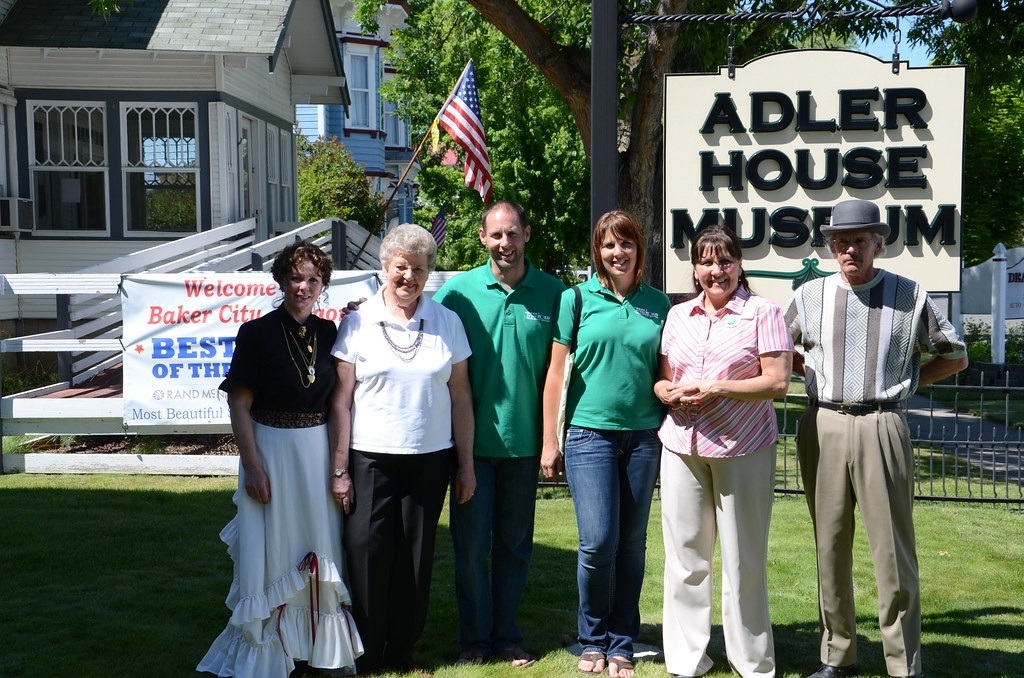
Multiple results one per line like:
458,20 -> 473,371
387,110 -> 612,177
329,469 -> 349,480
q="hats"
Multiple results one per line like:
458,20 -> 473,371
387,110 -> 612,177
819,198 -> 891,241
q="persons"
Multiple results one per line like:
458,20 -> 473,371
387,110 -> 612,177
341,197 -> 569,668
653,222 -> 794,678
215,239 -> 338,676
539,207 -> 671,678
328,221 -> 477,672
783,201 -> 968,677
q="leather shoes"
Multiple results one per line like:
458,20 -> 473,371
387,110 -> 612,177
798,661 -> 859,678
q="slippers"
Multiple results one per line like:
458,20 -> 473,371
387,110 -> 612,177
457,644 -> 537,669
579,652 -> 635,678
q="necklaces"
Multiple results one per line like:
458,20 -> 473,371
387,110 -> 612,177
379,319 -> 426,362
280,324 -> 318,388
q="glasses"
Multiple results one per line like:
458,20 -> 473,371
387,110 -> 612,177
831,236 -> 878,248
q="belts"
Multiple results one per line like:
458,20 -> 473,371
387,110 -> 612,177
807,398 -> 903,413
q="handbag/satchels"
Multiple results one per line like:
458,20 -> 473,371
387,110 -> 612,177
555,284 -> 582,477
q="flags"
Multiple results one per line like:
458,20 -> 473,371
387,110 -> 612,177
439,63 -> 492,205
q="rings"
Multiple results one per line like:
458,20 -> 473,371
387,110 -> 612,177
471,492 -> 474,495
